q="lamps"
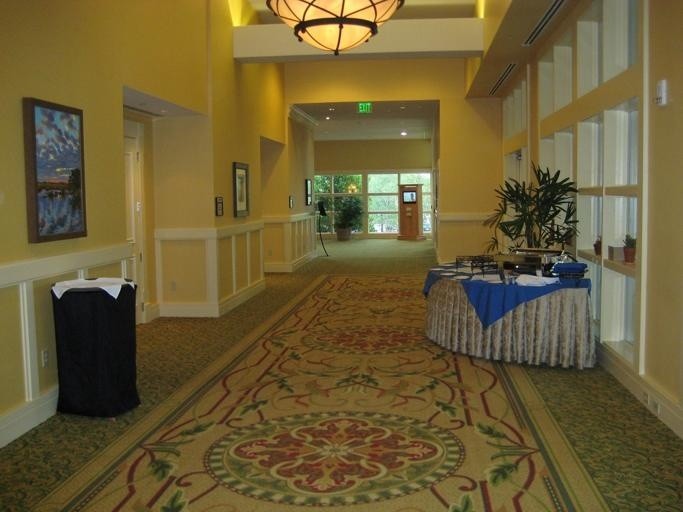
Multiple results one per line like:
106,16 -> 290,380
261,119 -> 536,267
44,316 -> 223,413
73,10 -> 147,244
266,0 -> 405,54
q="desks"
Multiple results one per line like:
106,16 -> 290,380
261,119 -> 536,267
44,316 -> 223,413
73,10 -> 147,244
425,257 -> 593,363
51,279 -> 138,418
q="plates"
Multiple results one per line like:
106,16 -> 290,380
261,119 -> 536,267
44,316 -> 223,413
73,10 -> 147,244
432,257 -> 474,280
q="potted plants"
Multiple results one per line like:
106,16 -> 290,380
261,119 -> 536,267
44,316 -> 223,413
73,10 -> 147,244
622,235 -> 637,262
334,194 -> 363,242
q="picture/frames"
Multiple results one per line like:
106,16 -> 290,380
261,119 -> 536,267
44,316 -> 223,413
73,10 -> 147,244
232,161 -> 250,216
22,95 -> 89,243
